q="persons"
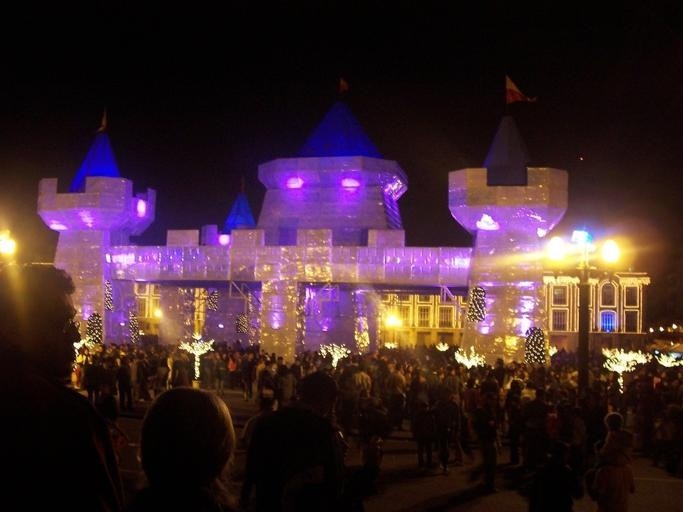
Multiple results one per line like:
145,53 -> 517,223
1,263 -> 124,511
73,338 -> 680,509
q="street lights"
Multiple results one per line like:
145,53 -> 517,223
550,232 -> 623,405
